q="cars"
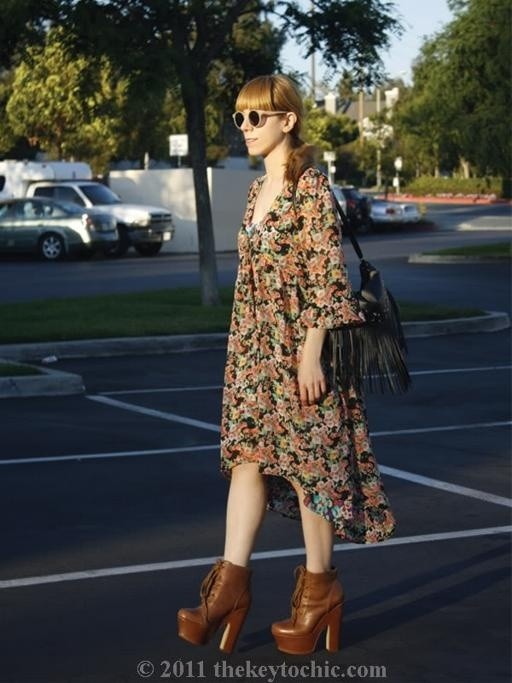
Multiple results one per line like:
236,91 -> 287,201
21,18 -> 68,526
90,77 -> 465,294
330,184 -> 422,235
0,181 -> 175,263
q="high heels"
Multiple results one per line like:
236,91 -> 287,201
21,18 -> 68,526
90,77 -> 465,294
272,566 -> 348,654
176,559 -> 253,654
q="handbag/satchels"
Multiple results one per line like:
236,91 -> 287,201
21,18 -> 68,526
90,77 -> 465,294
324,261 -> 411,396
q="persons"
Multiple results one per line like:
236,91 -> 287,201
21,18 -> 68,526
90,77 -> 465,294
176,74 -> 397,655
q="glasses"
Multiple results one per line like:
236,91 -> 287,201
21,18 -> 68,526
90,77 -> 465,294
233,110 -> 290,128
